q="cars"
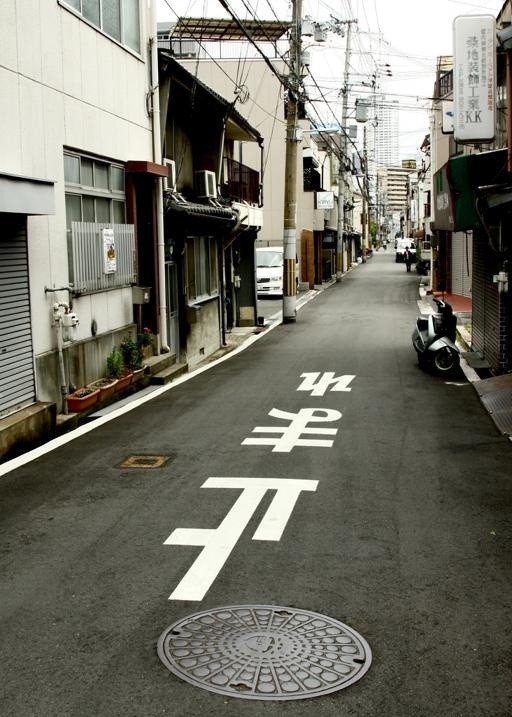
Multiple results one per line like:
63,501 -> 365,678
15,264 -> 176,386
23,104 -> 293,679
394,228 -> 417,262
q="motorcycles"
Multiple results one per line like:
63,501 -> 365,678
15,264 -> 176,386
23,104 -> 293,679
407,276 -> 464,377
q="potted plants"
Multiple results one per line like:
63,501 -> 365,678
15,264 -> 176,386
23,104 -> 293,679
104,345 -> 133,392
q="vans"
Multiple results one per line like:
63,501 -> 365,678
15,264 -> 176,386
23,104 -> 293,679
255,246 -> 301,298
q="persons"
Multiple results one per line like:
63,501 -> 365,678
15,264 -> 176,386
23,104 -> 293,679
403,246 -> 412,272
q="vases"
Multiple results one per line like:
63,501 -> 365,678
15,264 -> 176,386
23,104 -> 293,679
130,365 -> 148,382
64,388 -> 101,412
86,377 -> 118,401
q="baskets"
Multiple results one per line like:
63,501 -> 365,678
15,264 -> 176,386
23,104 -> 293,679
432,314 -> 457,334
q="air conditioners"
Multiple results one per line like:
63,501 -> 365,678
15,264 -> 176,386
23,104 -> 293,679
194,168 -> 219,200
162,153 -> 178,193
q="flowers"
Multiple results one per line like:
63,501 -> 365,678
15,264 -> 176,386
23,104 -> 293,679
120,322 -> 151,371
